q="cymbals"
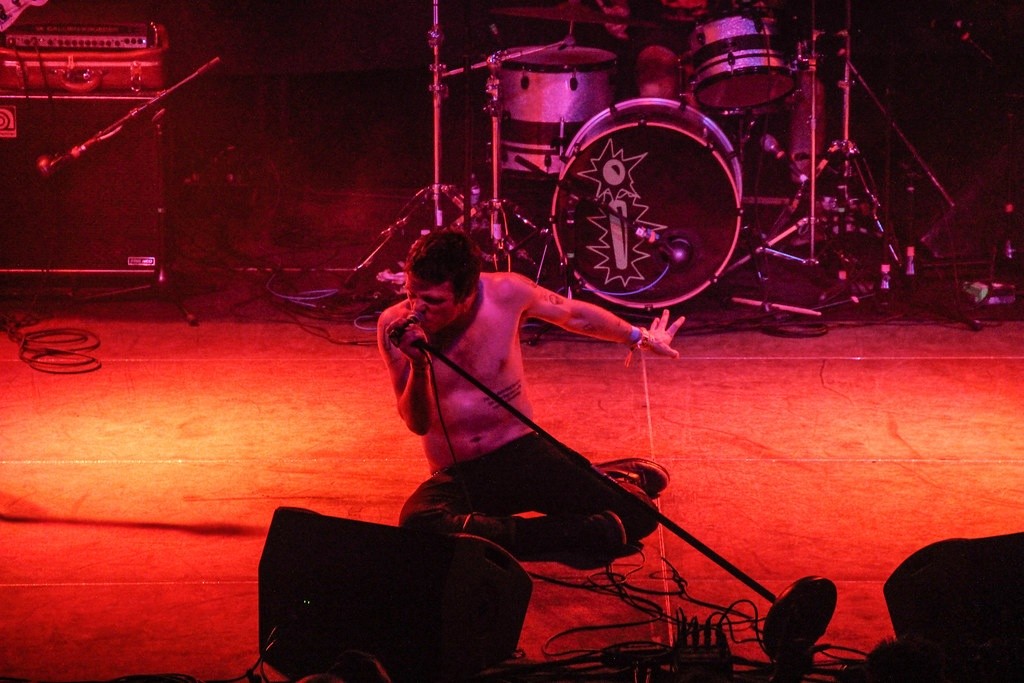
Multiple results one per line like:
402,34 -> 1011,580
491,0 -> 658,27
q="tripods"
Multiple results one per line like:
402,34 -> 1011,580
720,0 -> 1024,329
341,0 -> 549,324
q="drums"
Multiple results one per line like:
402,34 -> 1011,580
686,8 -> 801,113
548,98 -> 745,312
483,45 -> 618,183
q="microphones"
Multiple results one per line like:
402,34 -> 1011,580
391,310 -> 422,344
635,224 -> 694,267
35,143 -> 83,182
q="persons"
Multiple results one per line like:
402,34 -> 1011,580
376,226 -> 691,562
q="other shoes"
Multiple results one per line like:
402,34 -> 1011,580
597,458 -> 670,495
573,511 -> 625,557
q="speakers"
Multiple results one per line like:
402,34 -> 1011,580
0,88 -> 174,293
883,532 -> 1024,644
258,508 -> 534,683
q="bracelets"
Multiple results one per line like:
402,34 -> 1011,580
626,326 -> 655,353
